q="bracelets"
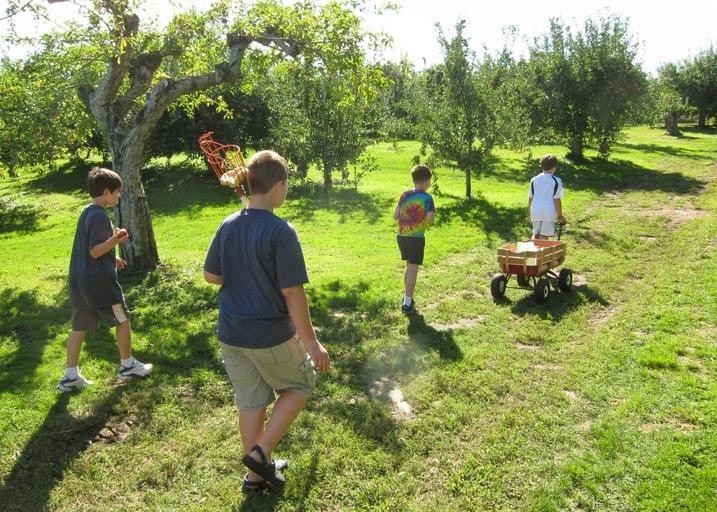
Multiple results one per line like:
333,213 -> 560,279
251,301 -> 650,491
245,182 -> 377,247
558,216 -> 564,218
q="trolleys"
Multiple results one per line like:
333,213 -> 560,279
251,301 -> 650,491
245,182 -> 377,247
488,222 -> 573,303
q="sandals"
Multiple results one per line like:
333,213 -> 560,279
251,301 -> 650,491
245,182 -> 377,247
242,460 -> 289,494
242,444 -> 285,487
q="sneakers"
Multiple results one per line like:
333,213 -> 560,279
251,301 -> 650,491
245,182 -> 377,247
55,375 -> 94,392
119,358 -> 153,379
402,298 -> 419,314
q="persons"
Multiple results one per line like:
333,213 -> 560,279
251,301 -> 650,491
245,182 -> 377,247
527,154 -> 565,240
201,149 -> 331,496
54,166 -> 157,392
598,137 -> 610,158
393,164 -> 436,312
